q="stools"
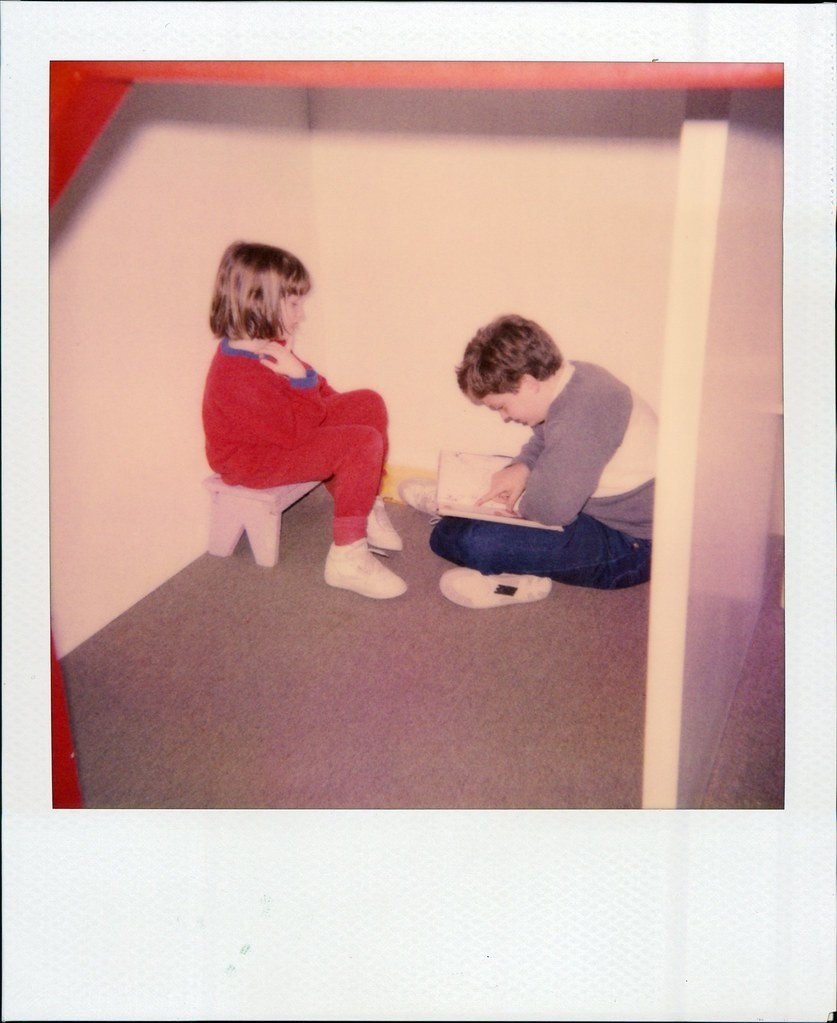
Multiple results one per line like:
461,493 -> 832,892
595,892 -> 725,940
202,473 -> 322,569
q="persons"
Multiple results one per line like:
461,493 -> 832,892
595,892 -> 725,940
203,243 -> 408,599
399,316 -> 660,608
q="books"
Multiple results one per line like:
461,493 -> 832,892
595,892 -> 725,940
436,451 -> 563,532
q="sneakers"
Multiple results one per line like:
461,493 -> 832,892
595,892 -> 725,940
367,495 -> 402,551
397,478 -> 445,524
323,537 -> 408,599
437,567 -> 552,610
